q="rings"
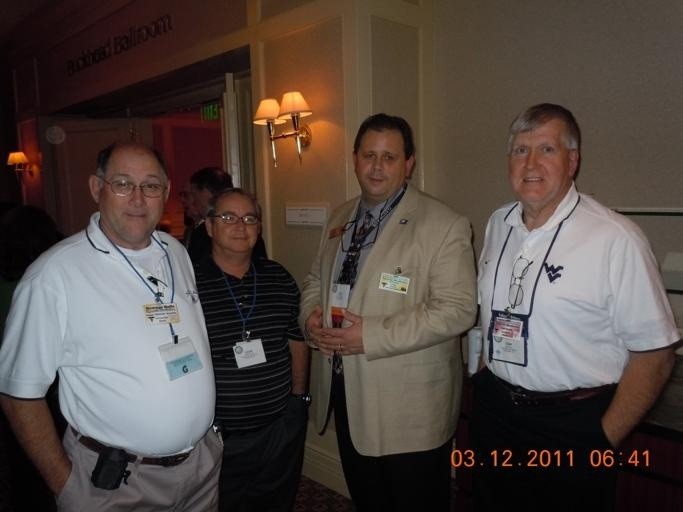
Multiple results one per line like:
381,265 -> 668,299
338,344 -> 342,351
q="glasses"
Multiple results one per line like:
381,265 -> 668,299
93,172 -> 170,199
333,213 -> 381,375
212,212 -> 263,225
508,253 -> 533,309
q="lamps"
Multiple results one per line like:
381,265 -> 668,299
252,91 -> 313,168
7,152 -> 32,180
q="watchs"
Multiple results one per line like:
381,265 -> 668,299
290,393 -> 311,405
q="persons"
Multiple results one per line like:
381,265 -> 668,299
0,204 -> 67,512
184,167 -> 268,258
470,103 -> 682,512
191,187 -> 311,512
0,139 -> 225,512
297,113 -> 478,512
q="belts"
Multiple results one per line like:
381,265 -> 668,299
69,425 -> 191,470
484,366 -> 616,412
